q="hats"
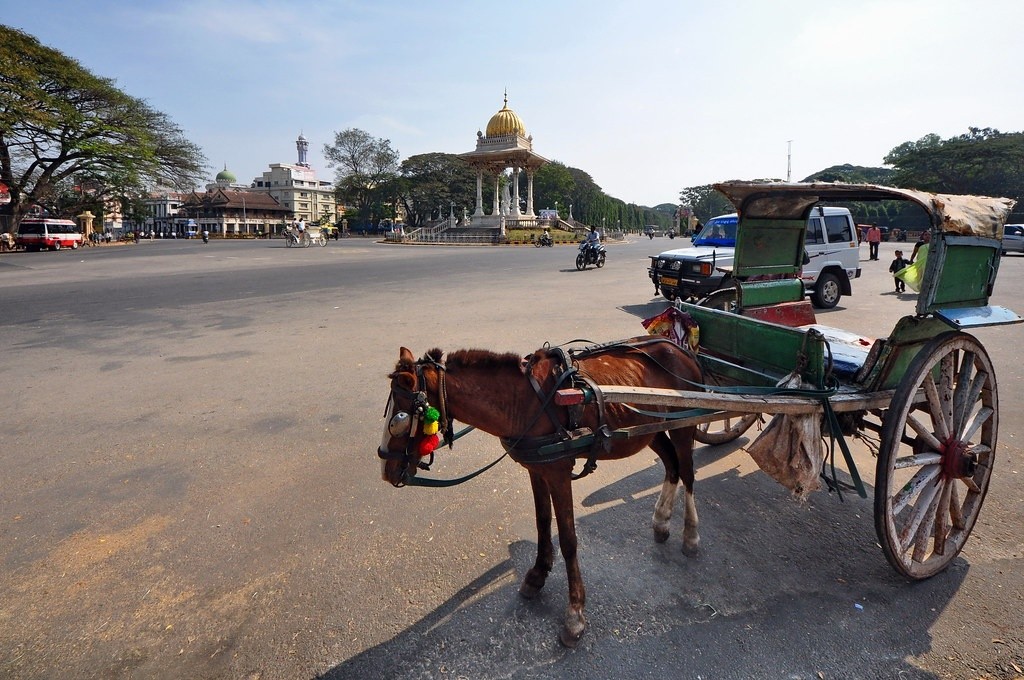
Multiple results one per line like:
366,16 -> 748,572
871,222 -> 878,227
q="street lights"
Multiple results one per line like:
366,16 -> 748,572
569,204 -> 572,220
602,218 -> 605,235
555,201 -> 558,218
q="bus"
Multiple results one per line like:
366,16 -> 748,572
16,218 -> 83,252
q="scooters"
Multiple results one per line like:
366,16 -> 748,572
576,240 -> 607,271
897,230 -> 907,242
203,238 -> 209,244
649,234 -> 654,239
669,233 -> 675,239
535,234 -> 554,248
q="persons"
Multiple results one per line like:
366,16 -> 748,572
542,228 -> 549,244
695,222 -> 703,235
88,229 -> 183,247
292,217 -> 306,241
669,227 -> 675,235
888,230 -> 931,294
648,227 -> 655,237
203,229 -> 210,243
854,222 -> 862,245
866,222 -> 881,261
0,230 -> 19,251
585,225 -> 600,264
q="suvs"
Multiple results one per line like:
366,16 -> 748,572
648,204 -> 862,309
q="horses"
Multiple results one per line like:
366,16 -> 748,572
375,336 -> 706,647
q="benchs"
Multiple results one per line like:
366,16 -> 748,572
789,324 -> 879,376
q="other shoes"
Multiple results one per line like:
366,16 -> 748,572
902,288 -> 905,292
875,258 -> 879,261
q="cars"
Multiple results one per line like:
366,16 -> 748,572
1001,224 -> 1024,255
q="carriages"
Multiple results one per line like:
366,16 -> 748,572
377,182 -> 1024,649
283,223 -> 327,248
